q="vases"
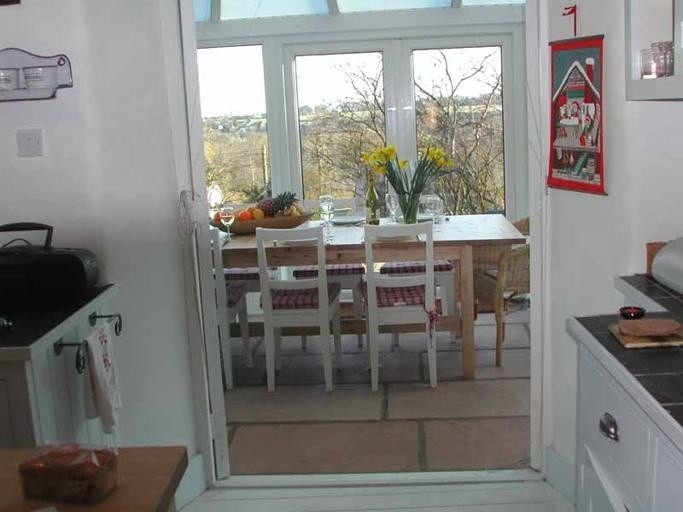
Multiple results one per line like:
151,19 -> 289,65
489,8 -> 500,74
358,145 -> 458,224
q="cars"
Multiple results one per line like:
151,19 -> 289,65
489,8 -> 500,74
638,41 -> 674,78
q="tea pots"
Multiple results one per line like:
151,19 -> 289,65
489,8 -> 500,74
621,1 -> 683,104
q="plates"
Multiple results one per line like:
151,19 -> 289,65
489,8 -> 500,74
568,337 -> 682,510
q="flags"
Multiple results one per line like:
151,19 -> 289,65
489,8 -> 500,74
397,190 -> 422,223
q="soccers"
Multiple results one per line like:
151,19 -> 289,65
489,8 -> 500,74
214,212 -> 222,223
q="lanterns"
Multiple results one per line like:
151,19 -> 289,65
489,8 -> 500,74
245,207 -> 264,220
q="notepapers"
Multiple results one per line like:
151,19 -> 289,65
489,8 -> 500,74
255,191 -> 299,217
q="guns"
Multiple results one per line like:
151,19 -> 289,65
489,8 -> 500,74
283,201 -> 305,217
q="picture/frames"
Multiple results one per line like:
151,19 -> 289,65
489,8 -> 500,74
0,445 -> 191,512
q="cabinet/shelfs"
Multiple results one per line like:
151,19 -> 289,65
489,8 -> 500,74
364,176 -> 381,225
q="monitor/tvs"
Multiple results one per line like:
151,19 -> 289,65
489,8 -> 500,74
608,324 -> 683,349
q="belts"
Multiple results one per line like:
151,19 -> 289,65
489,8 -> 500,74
220,207 -> 234,243
384,194 -> 443,234
318,195 -> 335,237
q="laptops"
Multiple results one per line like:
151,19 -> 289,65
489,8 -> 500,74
18,450 -> 118,504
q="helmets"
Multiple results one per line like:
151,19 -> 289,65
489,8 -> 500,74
285,238 -> 319,243
329,217 -> 364,225
375,233 -> 410,241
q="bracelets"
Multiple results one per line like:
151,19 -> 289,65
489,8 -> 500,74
620,306 -> 645,318
0,65 -> 59,92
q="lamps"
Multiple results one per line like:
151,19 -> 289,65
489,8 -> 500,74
460,217 -> 529,366
208,226 -> 252,391
354,220 -> 439,392
288,198 -> 367,354
223,202 -> 286,370
253,224 -> 343,393
381,195 -> 456,349
